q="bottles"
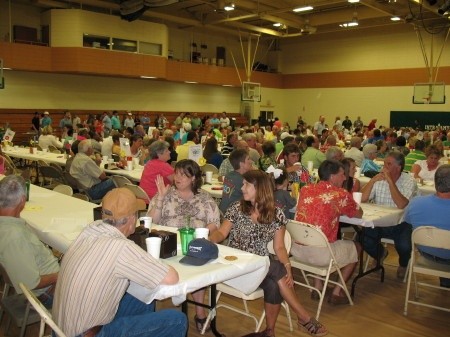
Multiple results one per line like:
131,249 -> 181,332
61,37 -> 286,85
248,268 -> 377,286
25,176 -> 30,201
126,157 -> 132,170
311,173 -> 317,183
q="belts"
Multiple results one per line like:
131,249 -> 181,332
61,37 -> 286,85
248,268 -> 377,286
39,284 -> 56,302
83,325 -> 102,337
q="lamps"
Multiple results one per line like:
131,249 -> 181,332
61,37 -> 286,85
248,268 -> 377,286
348,4 -> 359,27
224,0 -> 238,11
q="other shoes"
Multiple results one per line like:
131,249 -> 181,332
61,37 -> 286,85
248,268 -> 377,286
328,294 -> 350,304
311,289 -> 324,299
368,249 -> 388,269
194,312 -> 210,331
397,264 -> 408,279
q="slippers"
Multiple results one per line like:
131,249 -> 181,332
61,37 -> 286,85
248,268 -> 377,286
296,315 -> 327,336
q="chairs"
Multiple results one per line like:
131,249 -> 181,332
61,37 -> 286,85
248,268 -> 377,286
0,142 -> 450,337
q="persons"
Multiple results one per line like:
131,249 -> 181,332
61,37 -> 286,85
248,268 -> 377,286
207,170 -> 328,337
52,186 -> 188,337
29,110 -> 450,305
145,159 -> 220,332
0,174 -> 59,310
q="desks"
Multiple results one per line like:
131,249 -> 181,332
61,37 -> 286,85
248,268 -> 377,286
0,141 -> 407,232
0,172 -> 270,307
303,161 -> 436,201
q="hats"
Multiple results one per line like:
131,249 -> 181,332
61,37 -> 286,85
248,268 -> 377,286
180,239 -> 218,266
281,132 -> 293,139
101,188 -> 146,220
128,113 -> 132,115
43,112 -> 49,115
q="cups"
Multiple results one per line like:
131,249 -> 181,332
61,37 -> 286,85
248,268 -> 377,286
61,138 -> 65,143
206,171 -> 213,184
146,237 -> 161,260
102,156 -> 108,164
293,162 -> 302,177
195,228 -> 209,239
5,145 -> 10,151
14,146 -> 18,150
133,158 -> 139,168
179,227 -> 195,255
353,192 -> 362,205
307,161 -> 313,171
33,148 -> 37,155
139,217 -> 153,234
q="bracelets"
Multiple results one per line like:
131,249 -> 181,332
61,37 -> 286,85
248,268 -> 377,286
58,253 -> 64,262
154,205 -> 163,210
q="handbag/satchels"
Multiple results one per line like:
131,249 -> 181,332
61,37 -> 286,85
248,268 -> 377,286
364,171 -> 380,177
